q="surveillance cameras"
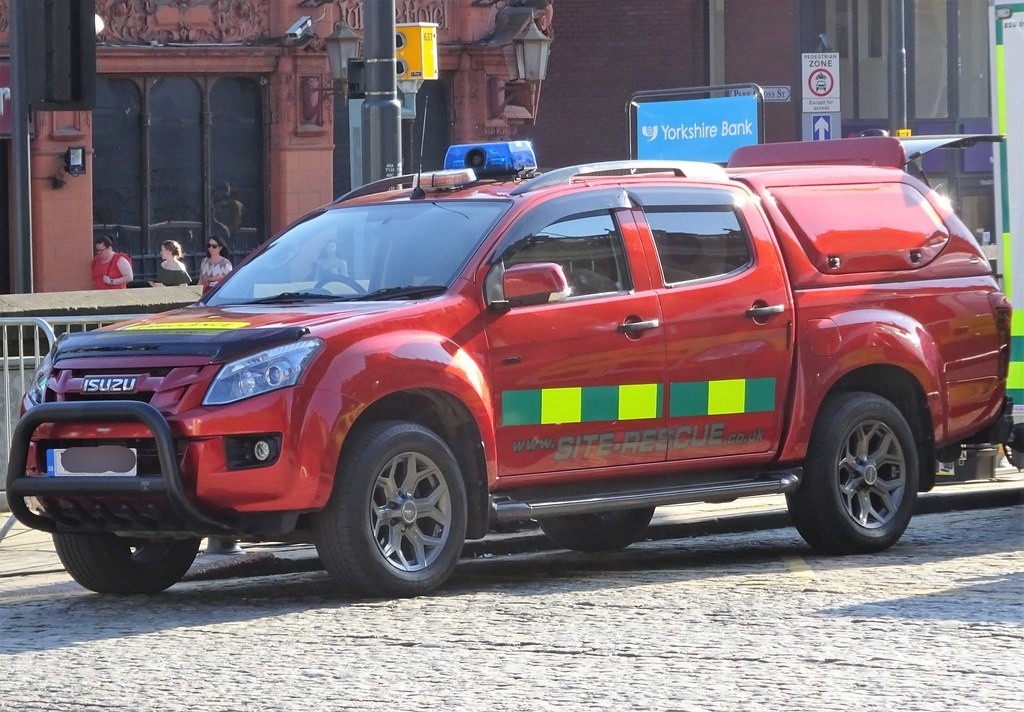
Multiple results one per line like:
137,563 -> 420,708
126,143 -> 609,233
286,15 -> 312,39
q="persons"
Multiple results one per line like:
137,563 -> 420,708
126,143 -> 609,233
314,238 -> 348,284
147,239 -> 193,287
91,233 -> 134,290
195,235 -> 233,293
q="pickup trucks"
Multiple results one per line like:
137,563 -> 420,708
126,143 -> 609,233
2,131 -> 1013,598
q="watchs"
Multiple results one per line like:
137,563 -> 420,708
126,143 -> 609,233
111,279 -> 114,285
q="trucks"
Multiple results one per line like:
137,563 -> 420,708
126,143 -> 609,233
972,0 -> 1024,431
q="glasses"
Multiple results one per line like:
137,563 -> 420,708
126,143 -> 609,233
96,245 -> 109,253
207,243 -> 219,249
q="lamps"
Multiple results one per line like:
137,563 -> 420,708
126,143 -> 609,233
300,15 -> 362,120
514,9 -> 552,105
63,146 -> 86,177
